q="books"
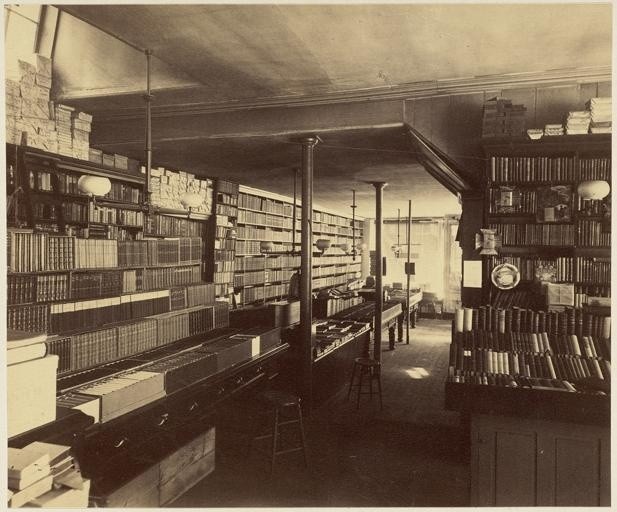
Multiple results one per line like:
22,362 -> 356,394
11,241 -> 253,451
8,53 -> 279,506
482,98 -> 613,138
217,180 -> 302,309
451,289 -> 611,394
489,220 -> 610,284
270,292 -> 375,357
491,156 -> 611,217
313,211 -> 363,289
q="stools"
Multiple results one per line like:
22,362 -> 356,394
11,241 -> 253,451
243,389 -> 311,479
345,358 -> 382,411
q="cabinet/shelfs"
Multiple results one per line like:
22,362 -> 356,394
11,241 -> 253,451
468,412 -> 611,507
444,134 -> 611,426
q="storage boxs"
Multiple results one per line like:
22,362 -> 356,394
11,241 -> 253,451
88,422 -> 217,508
5,353 -> 60,441
